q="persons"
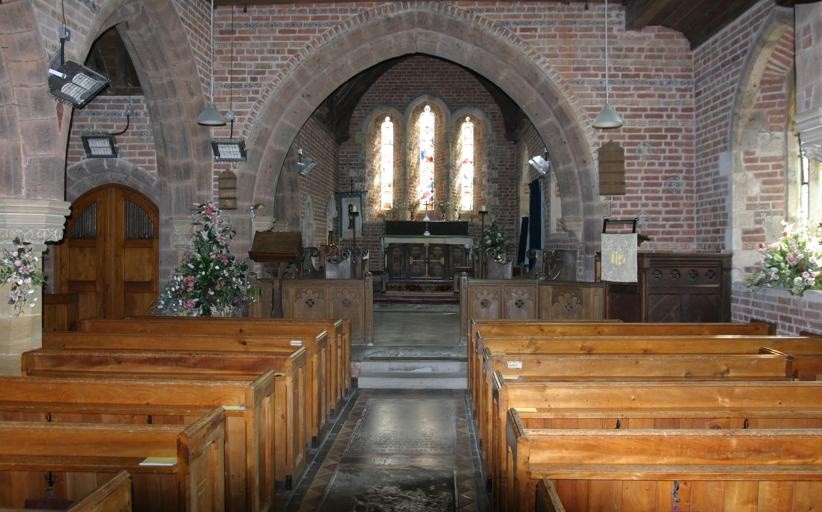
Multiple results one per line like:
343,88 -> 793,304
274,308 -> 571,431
348,204 -> 353,229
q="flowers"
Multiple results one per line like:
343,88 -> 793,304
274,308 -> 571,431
0,230 -> 49,317
475,217 -> 514,260
743,212 -> 822,298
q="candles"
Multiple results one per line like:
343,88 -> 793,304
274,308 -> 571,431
353,205 -> 356,212
480,205 -> 485,211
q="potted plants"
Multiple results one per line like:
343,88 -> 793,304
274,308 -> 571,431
439,198 -> 459,221
395,195 -> 420,222
376,205 -> 396,221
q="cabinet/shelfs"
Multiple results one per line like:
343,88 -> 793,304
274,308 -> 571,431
606,253 -> 731,323
381,234 -> 474,292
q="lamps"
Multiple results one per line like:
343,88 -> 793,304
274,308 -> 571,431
48,0 -> 111,108
294,136 -> 316,177
211,1 -> 247,163
80,105 -> 133,158
592,0 -> 623,129
528,147 -> 548,181
196,0 -> 225,127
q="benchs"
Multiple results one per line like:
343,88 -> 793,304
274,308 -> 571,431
466,319 -> 822,512
1,318 -> 352,512
